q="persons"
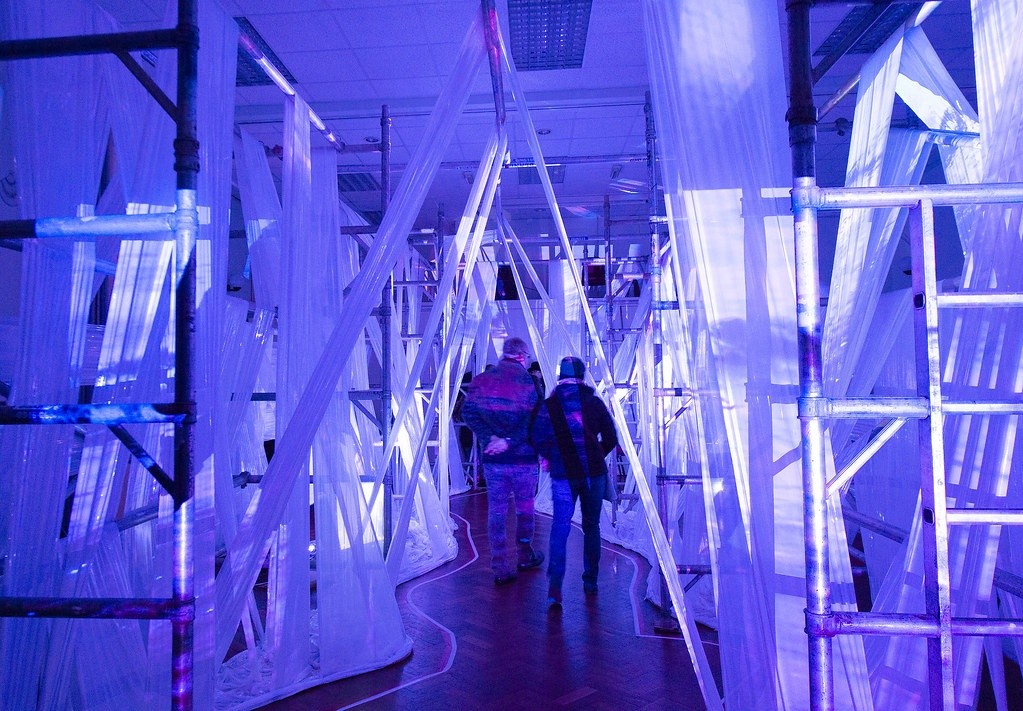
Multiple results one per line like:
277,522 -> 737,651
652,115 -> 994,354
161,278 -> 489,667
529,353 -> 619,618
453,338 -> 546,580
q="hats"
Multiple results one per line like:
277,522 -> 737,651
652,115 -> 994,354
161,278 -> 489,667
527,361 -> 540,372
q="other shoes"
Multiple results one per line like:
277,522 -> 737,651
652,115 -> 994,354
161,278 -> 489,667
548,593 -> 563,608
517,550 -> 545,569
477,479 -> 487,487
465,478 -> 473,485
495,568 -> 517,585
584,581 -> 600,592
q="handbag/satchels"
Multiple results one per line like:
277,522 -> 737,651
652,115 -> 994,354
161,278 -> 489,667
599,442 -> 618,502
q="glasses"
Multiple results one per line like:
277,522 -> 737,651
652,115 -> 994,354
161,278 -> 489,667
523,349 -> 531,359
